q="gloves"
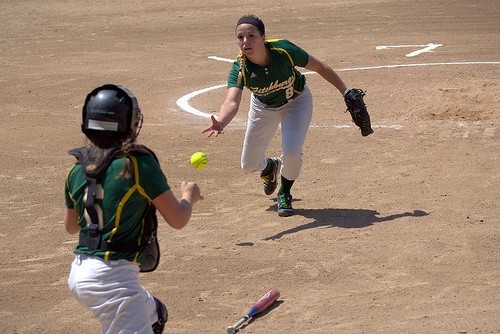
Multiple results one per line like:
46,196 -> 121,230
344,87 -> 374,136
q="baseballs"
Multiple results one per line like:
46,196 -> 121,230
191,151 -> 208,170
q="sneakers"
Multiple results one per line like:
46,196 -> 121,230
277,188 -> 294,217
259,157 -> 282,195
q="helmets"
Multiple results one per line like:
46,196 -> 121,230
80,83 -> 143,146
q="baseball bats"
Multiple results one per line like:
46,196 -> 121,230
227,289 -> 281,334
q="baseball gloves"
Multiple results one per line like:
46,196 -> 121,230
343,88 -> 374,137
